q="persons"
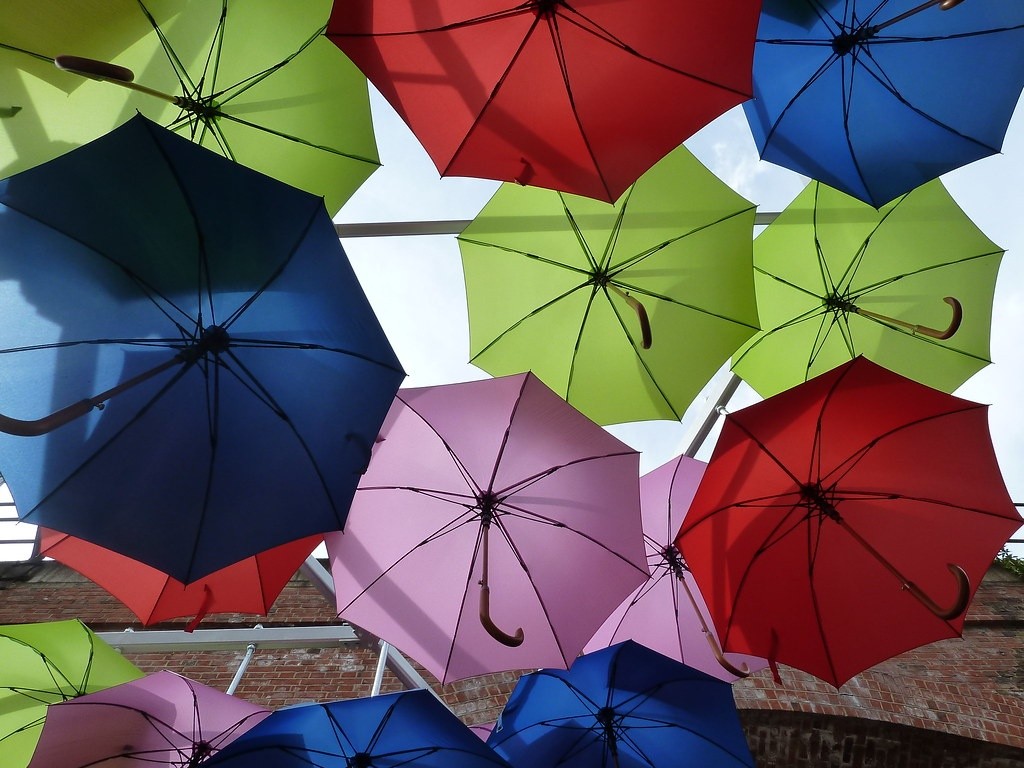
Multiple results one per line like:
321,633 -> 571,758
0,0 -> 381,227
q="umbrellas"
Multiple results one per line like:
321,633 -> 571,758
669,353 -> 1024,695
740,1 -> 1024,213
0,113 -> 409,590
323,0 -> 760,206
0,369 -> 758,768
728,173 -> 1008,402
455,134 -> 763,429
